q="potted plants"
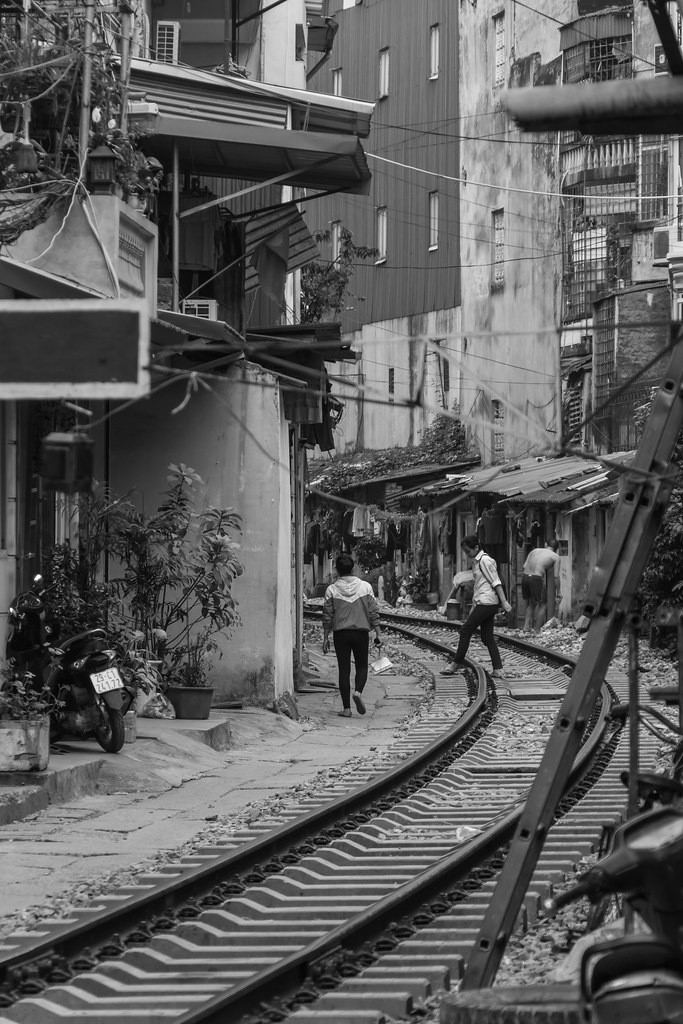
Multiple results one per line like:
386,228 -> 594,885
154,461 -> 246,720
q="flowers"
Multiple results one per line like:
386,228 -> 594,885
0,645 -> 72,724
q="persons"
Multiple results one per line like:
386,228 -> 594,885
438,536 -> 511,677
521,539 -> 565,634
322,556 -> 381,716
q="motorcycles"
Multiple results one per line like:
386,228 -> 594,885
541,798 -> 683,1023
0,575 -> 135,753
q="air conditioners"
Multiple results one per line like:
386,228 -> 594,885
183,298 -> 218,320
155,20 -> 179,65
652,225 -> 678,265
654,44 -> 668,76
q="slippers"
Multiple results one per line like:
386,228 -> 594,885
490,672 -> 502,678
440,668 -> 459,675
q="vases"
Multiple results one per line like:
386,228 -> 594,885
0,715 -> 52,772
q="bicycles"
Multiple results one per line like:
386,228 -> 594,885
584,704 -> 683,932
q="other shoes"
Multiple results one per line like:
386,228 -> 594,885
352,692 -> 366,715
338,711 -> 351,716
